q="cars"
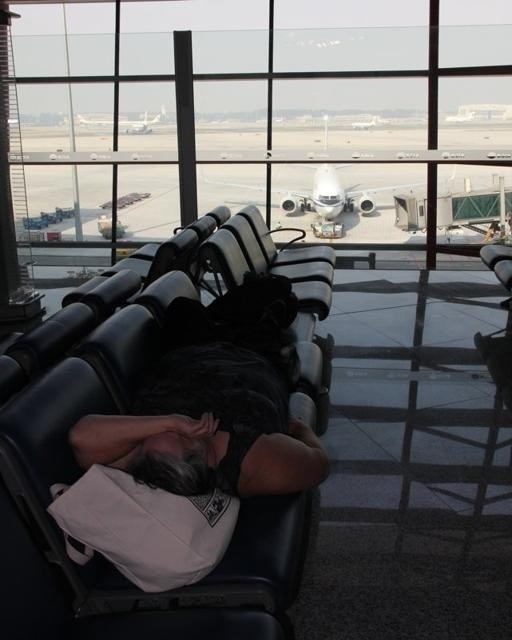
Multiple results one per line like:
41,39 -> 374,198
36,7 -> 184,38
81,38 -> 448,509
102,190 -> 151,209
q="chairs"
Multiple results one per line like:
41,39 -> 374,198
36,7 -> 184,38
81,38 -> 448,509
478,242 -> 512,290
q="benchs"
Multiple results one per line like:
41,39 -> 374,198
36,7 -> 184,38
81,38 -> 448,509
0,203 -> 339,638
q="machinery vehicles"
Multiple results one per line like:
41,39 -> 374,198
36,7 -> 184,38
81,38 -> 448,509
23,205 -> 76,229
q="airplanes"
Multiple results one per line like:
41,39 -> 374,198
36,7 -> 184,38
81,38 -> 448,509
445,112 -> 474,123
350,115 -> 378,130
204,153 -> 442,224
76,104 -> 171,135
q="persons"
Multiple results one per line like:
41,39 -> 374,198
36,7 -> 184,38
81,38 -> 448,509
276,221 -> 282,229
69,296 -> 331,496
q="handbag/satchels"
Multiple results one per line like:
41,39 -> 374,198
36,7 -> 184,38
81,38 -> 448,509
50,464 -> 240,593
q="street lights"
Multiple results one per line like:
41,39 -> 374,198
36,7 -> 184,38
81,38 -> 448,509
320,111 -> 330,153
